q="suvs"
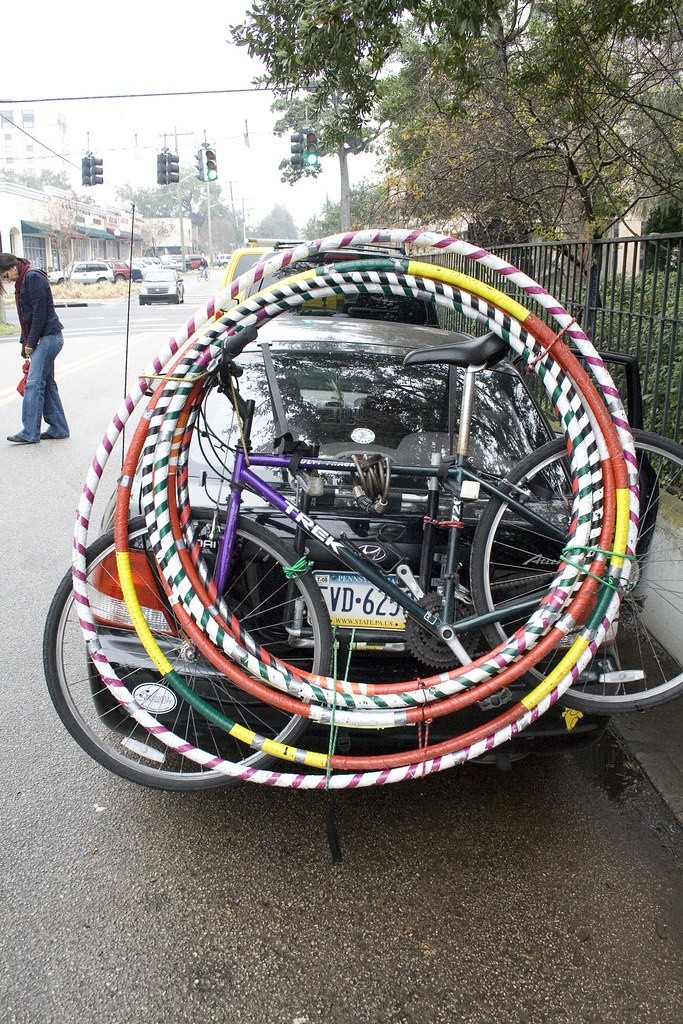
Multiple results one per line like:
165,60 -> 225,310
46,260 -> 116,286
243,241 -> 442,330
216,239 -> 346,310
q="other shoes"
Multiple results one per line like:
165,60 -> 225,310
40,431 -> 59,439
6,434 -> 37,445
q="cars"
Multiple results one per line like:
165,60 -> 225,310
95,255 -> 203,282
95,310 -> 661,767
135,269 -> 185,306
215,254 -> 231,267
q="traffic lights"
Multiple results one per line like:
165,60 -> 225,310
193,150 -> 205,183
305,131 -> 319,167
167,154 -> 180,184
290,134 -> 306,166
204,149 -> 218,181
89,156 -> 104,185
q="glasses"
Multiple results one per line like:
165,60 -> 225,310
2,269 -> 10,279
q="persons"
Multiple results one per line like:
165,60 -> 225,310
198,258 -> 208,279
0,253 -> 71,445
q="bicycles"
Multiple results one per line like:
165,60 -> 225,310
197,266 -> 211,283
40,327 -> 683,794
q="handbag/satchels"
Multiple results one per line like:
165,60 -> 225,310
16,352 -> 31,397
